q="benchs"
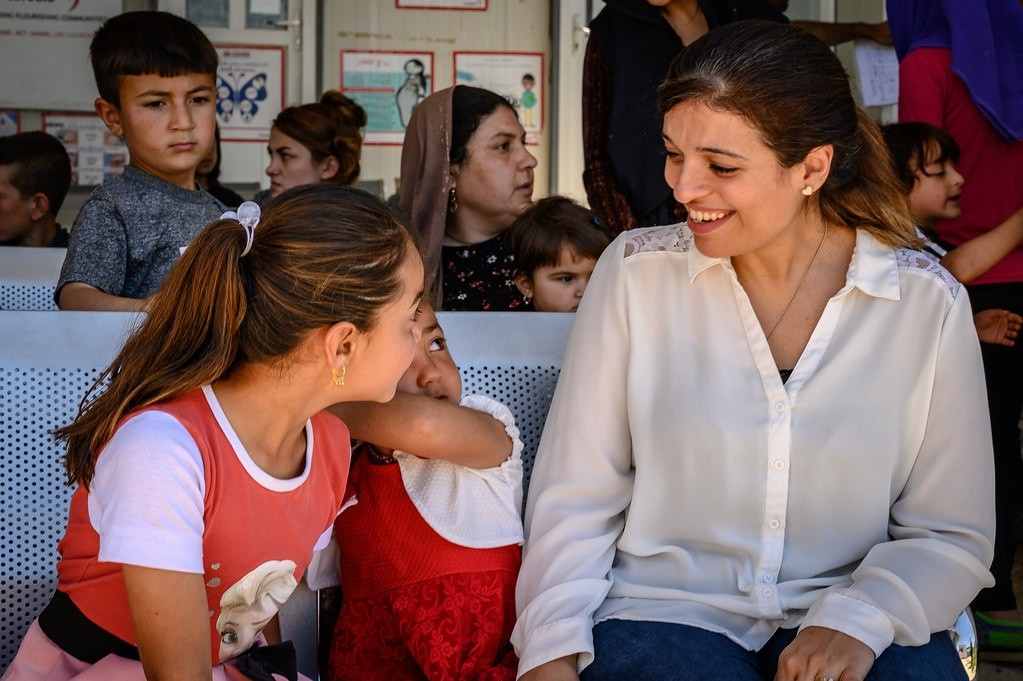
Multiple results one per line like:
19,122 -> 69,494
0,246 -> 577,679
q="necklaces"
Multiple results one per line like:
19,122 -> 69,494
445,232 -> 473,245
767,219 -> 828,340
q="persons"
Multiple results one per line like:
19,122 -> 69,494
266,89 -> 368,200
509,19 -> 996,681
886,1 -> 1023,613
307,298 -> 525,681
510,196 -> 610,312
877,122 -> 1023,665
54,10 -> 246,312
582,0 -> 894,243
0,184 -> 424,681
0,130 -> 72,248
389,85 -> 537,312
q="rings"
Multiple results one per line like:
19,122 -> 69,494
820,677 -> 834,681
